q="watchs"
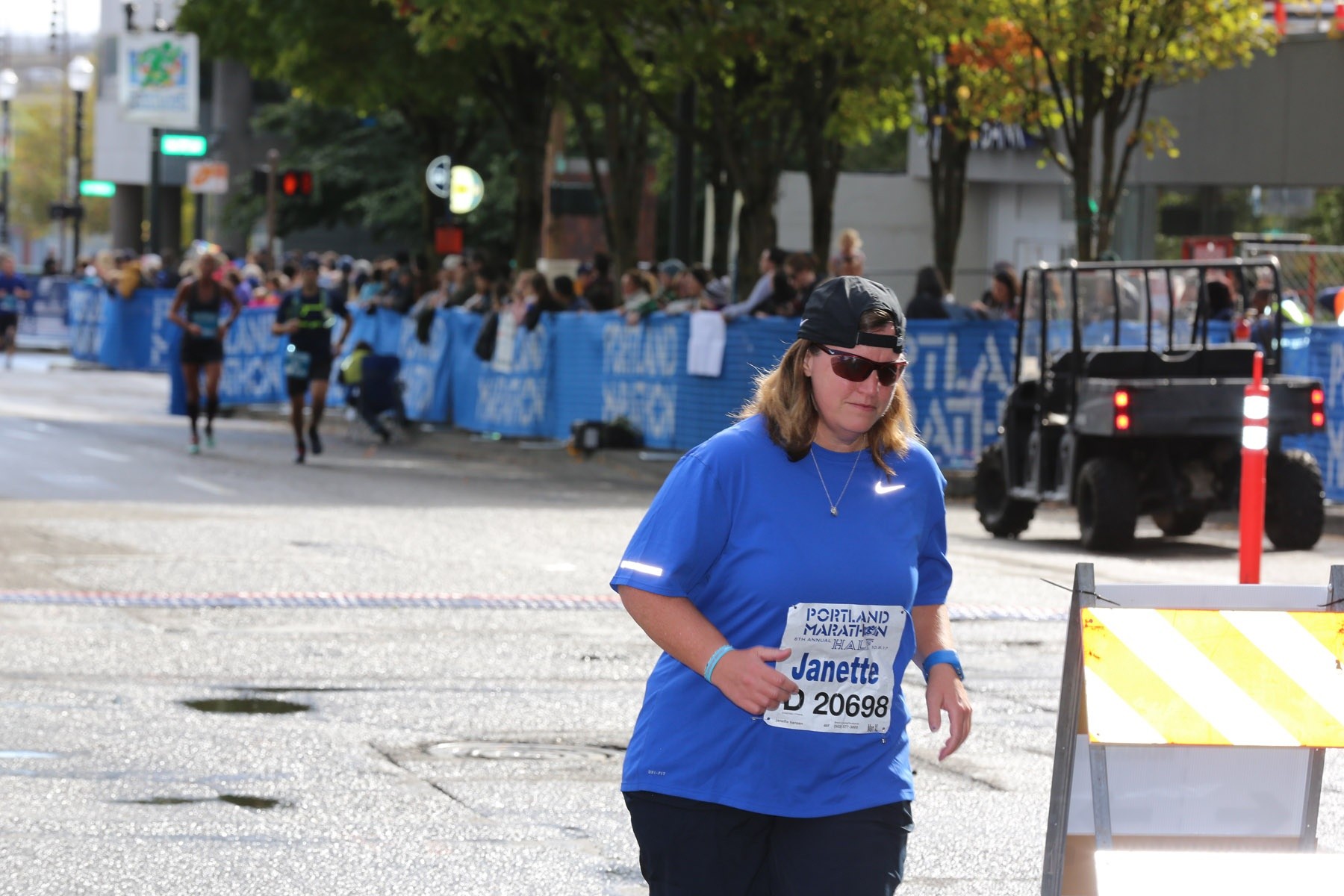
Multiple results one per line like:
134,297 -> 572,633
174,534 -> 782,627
922,649 -> 965,682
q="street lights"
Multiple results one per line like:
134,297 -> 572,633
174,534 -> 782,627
67,57 -> 94,275
0,71 -> 19,258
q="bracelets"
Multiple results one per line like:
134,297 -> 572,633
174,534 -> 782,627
704,645 -> 734,683
340,337 -> 344,343
227,322 -> 230,327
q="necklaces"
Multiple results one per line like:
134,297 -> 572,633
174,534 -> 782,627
810,438 -> 865,515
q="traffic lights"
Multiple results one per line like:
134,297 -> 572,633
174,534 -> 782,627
276,171 -> 312,210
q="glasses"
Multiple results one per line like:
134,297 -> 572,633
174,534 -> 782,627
815,341 -> 908,387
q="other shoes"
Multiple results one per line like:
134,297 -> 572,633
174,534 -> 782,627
190,441 -> 198,454
296,452 -> 305,464
308,425 -> 321,455
205,427 -> 213,447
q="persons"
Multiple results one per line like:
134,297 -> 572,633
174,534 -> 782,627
609,276 -> 972,896
272,258 -> 354,463
0,228 -> 1343,371
168,253 -> 242,456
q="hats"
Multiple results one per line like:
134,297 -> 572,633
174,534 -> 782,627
659,259 -> 686,281
799,276 -> 905,353
704,275 -> 731,304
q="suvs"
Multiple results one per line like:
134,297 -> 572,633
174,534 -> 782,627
973,257 -> 1326,551
